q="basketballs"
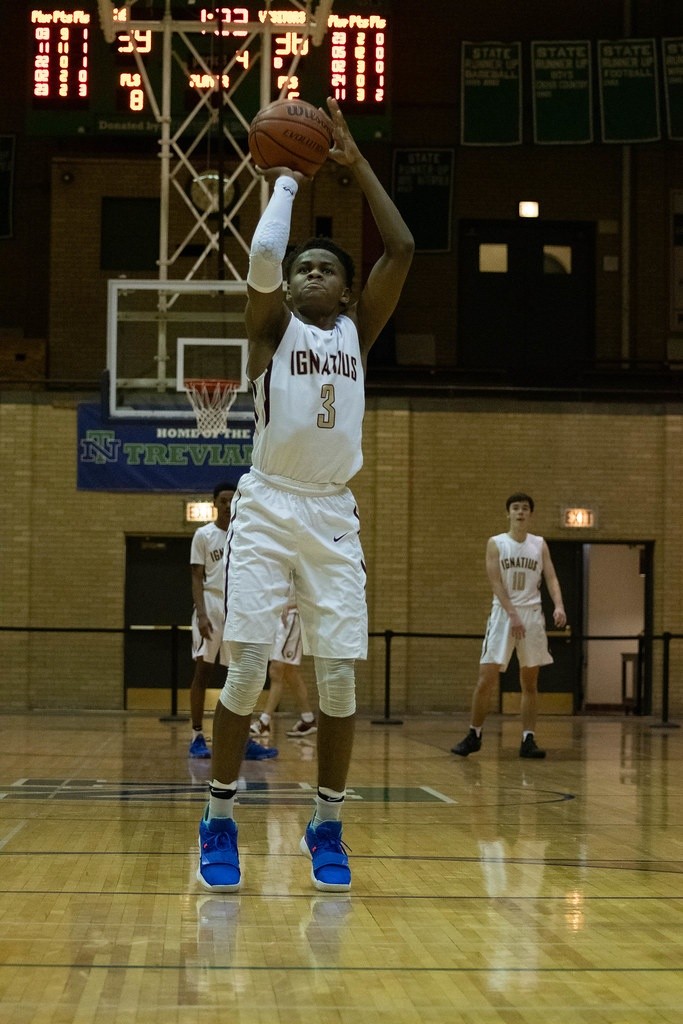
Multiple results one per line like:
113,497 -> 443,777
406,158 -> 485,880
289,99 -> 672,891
248,97 -> 333,181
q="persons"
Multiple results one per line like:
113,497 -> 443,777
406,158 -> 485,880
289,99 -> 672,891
188,483 -> 318,761
451,492 -> 567,759
195,96 -> 416,893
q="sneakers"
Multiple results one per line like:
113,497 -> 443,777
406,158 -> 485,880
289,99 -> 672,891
252,738 -> 269,750
465,761 -> 483,788
519,733 -> 546,759
189,759 -> 212,781
189,733 -> 212,759
196,893 -> 241,964
286,737 -> 317,756
247,717 -> 271,738
239,758 -> 280,776
243,739 -> 279,761
196,801 -> 241,892
299,806 -> 352,892
518,766 -> 535,786
298,891 -> 353,946
285,716 -> 317,737
451,728 -> 484,757
